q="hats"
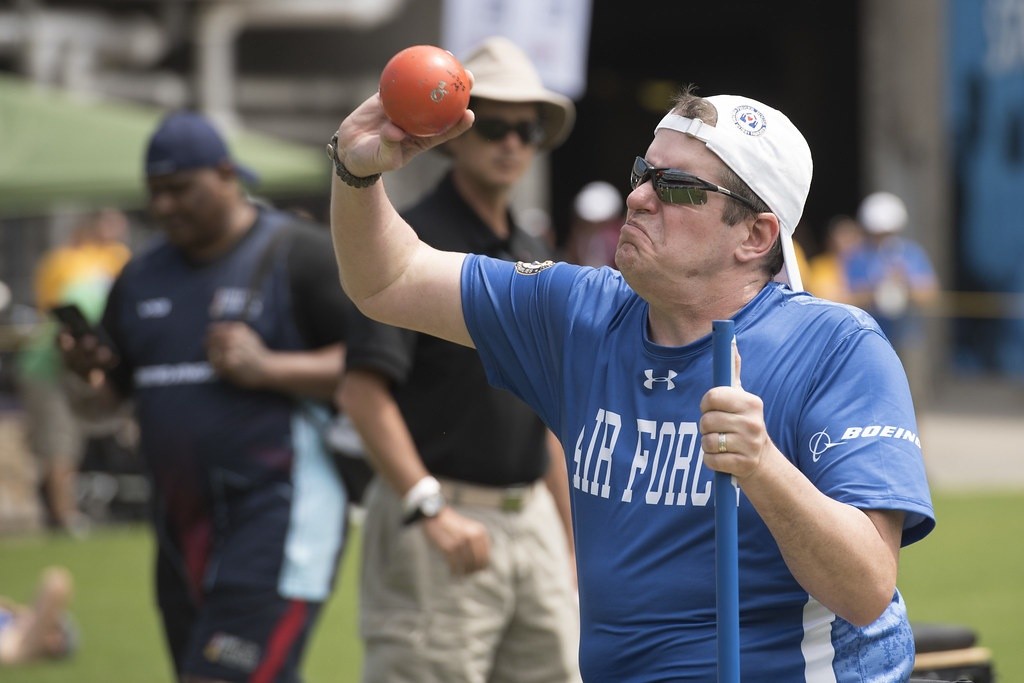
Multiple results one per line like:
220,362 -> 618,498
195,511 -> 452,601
462,36 -> 573,148
146,116 -> 225,171
655,94 -> 814,291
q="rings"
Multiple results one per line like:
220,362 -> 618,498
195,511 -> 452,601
717,432 -> 727,453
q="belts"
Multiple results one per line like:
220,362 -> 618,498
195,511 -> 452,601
440,479 -> 538,511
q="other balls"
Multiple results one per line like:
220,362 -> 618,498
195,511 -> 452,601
378,45 -> 472,137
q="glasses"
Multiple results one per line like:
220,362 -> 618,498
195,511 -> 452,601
475,117 -> 534,144
631,157 -> 763,215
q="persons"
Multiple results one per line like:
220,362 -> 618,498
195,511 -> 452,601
57,110 -> 356,683
340,37 -> 582,683
325,44 -> 937,683
0,0 -> 938,683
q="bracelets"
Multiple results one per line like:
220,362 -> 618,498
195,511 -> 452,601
401,491 -> 447,527
325,130 -> 384,189
399,475 -> 441,510
